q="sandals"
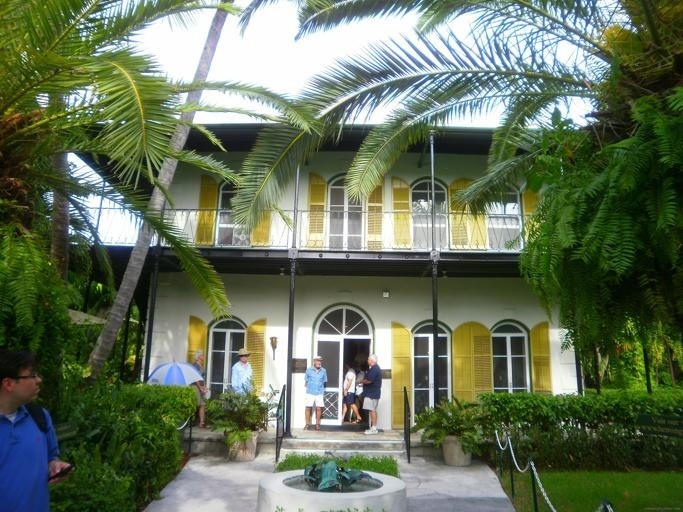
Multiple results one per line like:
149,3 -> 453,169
315,424 -> 321,430
352,416 -> 364,424
304,424 -> 309,430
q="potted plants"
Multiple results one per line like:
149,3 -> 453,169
200,383 -> 268,462
410,393 -> 492,467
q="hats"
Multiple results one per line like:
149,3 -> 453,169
313,356 -> 323,360
234,348 -> 251,358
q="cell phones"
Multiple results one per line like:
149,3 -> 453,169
48,467 -> 70,482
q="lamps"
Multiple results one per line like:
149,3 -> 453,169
270,336 -> 277,361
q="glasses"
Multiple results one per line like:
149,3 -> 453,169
9,371 -> 39,380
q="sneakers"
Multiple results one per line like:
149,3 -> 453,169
363,426 -> 378,435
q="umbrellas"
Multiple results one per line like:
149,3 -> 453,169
147,359 -> 205,388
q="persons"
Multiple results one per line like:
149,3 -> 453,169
304,356 -> 328,431
231,348 -> 252,395
342,360 -> 365,424
0,349 -> 72,512
357,354 -> 382,435
192,351 -> 209,428
